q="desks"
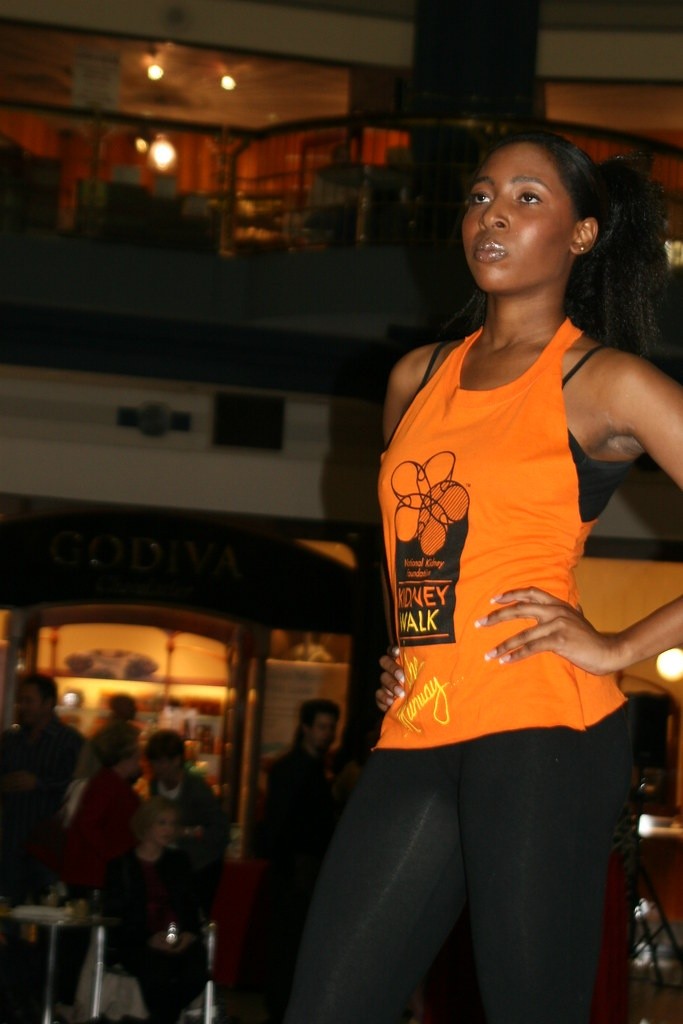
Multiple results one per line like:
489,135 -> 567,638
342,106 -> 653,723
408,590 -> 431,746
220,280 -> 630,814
0,907 -> 119,1024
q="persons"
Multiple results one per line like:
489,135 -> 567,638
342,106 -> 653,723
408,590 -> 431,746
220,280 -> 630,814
0,672 -> 84,944
103,795 -> 208,1024
91,696 -> 143,786
233,699 -> 341,1024
281,137 -> 683,1024
22,736 -> 138,1004
143,731 -> 228,862
